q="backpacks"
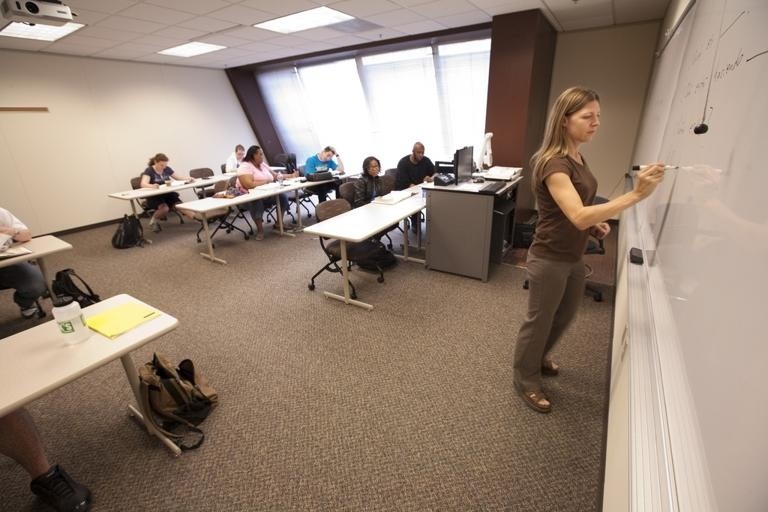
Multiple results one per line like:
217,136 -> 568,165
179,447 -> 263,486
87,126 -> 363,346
300,170 -> 339,184
50,268 -> 103,308
138,352 -> 219,450
112,213 -> 145,249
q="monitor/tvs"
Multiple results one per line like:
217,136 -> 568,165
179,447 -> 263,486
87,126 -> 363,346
454,145 -> 472,186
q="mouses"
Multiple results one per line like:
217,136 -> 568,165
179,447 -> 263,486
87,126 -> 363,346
474,178 -> 485,183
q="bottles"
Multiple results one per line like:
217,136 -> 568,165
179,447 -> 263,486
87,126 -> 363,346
52,294 -> 90,345
277,172 -> 284,189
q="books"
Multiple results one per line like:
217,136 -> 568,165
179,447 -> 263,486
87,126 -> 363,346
84,300 -> 162,342
0,233 -> 34,259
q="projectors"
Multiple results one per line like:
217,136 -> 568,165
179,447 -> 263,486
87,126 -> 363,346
2,0 -> 73,27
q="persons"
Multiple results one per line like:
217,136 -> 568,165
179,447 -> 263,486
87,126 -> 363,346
0,407 -> 91,510
140,152 -> 204,233
225,143 -> 246,174
352,156 -> 400,242
395,141 -> 438,234
510,84 -> 666,418
303,145 -> 345,204
235,144 -> 300,241
0,206 -> 48,320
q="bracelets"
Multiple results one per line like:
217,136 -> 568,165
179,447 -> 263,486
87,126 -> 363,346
335,154 -> 339,157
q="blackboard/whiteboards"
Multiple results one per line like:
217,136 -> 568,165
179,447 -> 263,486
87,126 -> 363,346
627,0 -> 768,512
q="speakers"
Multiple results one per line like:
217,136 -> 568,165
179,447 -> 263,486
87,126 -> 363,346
491,199 -> 516,259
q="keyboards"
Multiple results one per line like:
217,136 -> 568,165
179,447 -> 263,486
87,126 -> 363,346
479,182 -> 506,196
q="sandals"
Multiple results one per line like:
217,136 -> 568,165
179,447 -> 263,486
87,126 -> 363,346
513,372 -> 551,413
274,221 -> 294,230
542,359 -> 559,376
255,229 -> 265,241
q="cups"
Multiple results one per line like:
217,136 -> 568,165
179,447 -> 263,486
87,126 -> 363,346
165,181 -> 172,188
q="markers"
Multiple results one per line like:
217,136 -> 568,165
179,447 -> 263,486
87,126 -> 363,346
632,165 -> 679,171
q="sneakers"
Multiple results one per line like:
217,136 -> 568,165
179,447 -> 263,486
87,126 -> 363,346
149,221 -> 161,234
412,222 -> 418,235
17,298 -> 36,319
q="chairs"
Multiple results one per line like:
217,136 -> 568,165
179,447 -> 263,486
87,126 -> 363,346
523,194 -> 612,303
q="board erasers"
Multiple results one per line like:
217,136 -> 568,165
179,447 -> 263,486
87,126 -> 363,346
630,248 -> 643,264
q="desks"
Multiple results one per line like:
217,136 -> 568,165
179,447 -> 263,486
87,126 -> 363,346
1,294 -> 184,457
303,178 -> 437,312
0,235 -> 74,302
107,164 -> 287,245
421,171 -> 524,283
